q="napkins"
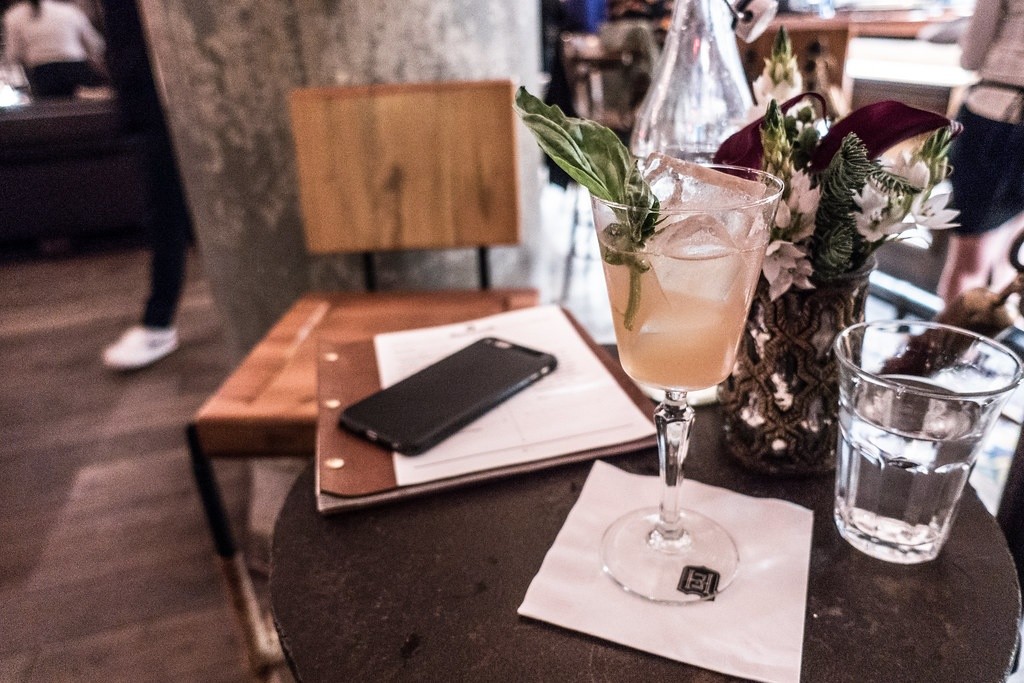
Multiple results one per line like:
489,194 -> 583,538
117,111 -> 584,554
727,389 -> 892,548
514,460 -> 817,682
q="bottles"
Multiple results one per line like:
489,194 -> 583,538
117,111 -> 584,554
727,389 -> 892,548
626,0 -> 758,170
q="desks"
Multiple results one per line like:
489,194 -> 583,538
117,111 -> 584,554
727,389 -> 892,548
267,342 -> 1024,683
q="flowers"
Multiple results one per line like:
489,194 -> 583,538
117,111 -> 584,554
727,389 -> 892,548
710,24 -> 967,278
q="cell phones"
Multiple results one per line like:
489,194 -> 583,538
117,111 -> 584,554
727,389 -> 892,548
338,337 -> 556,455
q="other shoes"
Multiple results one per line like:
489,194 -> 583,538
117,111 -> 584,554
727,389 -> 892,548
103,324 -> 180,371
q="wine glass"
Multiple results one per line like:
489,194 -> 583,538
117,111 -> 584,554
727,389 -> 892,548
587,162 -> 796,605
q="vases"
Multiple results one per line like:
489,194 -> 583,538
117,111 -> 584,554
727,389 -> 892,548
721,257 -> 881,478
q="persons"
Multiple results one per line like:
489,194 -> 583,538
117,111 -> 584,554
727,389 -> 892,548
936,0 -> 1024,366
542,0 -> 607,189
101,0 -> 200,369
0,0 -> 107,101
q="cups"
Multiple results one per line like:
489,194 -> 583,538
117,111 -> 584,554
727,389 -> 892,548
831,320 -> 1024,566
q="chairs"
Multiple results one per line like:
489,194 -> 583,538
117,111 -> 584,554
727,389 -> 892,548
181,77 -> 540,578
